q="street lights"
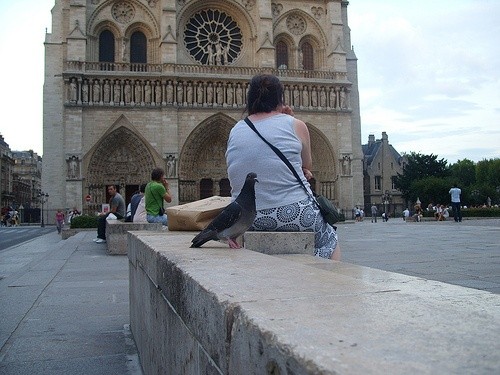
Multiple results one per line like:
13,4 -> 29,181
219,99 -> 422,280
381,189 -> 393,222
36,192 -> 50,228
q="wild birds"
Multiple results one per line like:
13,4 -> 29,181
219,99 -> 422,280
189,172 -> 260,248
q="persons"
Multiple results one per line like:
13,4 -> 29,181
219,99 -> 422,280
93,185 -> 125,243
434,203 -> 443,221
144,168 -> 172,226
412,205 -> 421,222
355,206 -> 361,222
125,183 -> 147,221
371,202 -> 377,223
0,210 -> 19,228
402,207 -> 409,222
381,210 -> 391,222
437,205 -> 449,221
56,209 -> 79,234
360,208 -> 364,220
448,184 -> 462,223
224,74 -> 340,260
487,196 -> 492,208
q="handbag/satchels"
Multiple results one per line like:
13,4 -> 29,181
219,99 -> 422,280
316,194 -> 340,226
158,208 -> 165,216
165,194 -> 235,231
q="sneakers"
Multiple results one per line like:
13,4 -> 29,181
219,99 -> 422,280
92,238 -> 107,243
